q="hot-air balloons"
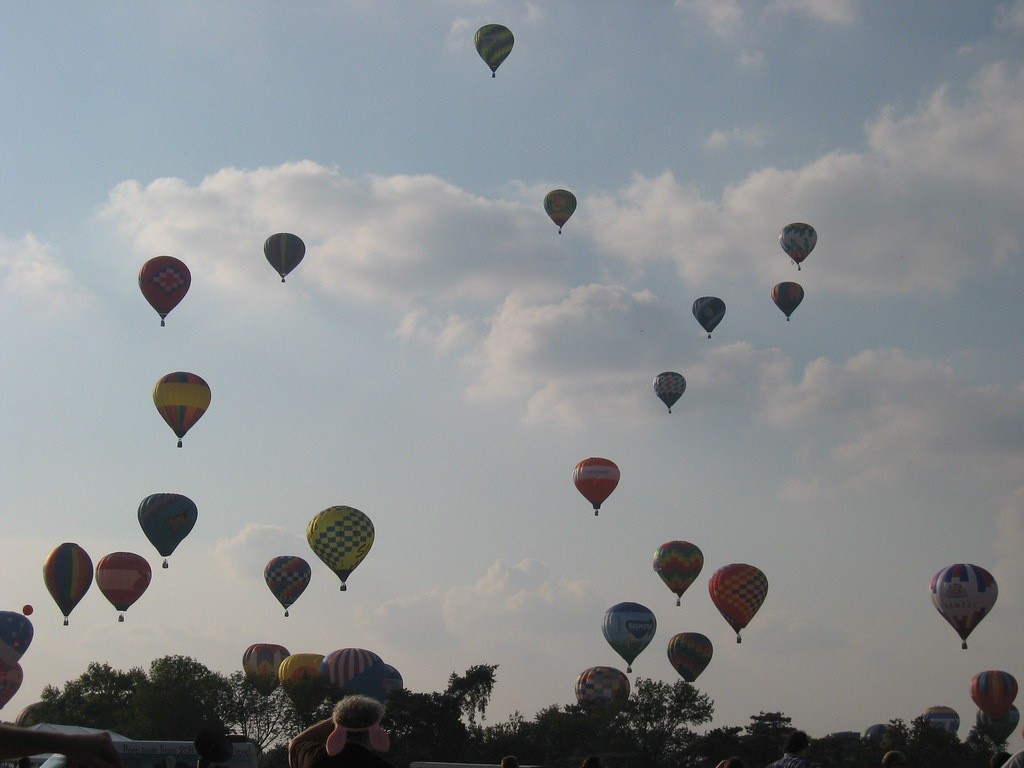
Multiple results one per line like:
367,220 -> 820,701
602,603 -> 657,672
152,371 -> 212,447
137,493 -> 197,569
474,24 -> 513,77
652,540 -> 703,606
0,605 -> 36,709
264,234 -> 306,282
771,222 -> 817,320
929,562 -> 999,650
864,670 -> 1020,768
43,542 -> 153,626
575,667 -> 632,739
543,190 -> 577,232
263,505 -> 375,617
574,458 -> 619,514
709,565 -> 768,644
668,631 -> 713,688
652,297 -> 726,413
138,256 -> 191,327
242,643 -> 404,724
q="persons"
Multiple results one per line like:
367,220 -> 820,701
715,757 -> 744,768
991,752 -> 1011,768
581,757 -> 601,768
501,756 -> 519,768
881,750 -> 908,768
0,727 -> 121,768
766,731 -> 810,768
287,695 -> 398,768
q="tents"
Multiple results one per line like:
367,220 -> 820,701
31,722 -> 132,741
